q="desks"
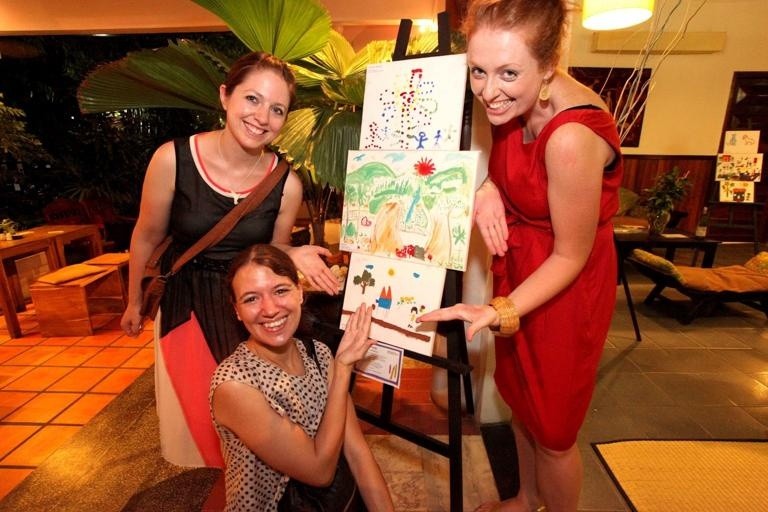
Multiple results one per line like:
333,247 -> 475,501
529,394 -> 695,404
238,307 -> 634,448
0,222 -> 109,339
613,229 -> 721,287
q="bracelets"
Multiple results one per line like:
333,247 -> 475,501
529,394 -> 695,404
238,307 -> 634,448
488,297 -> 520,338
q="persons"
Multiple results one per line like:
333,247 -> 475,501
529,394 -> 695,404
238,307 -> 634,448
415,0 -> 624,512
207,245 -> 395,512
119,52 -> 342,469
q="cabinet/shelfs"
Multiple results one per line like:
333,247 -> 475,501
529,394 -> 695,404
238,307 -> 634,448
700,69 -> 768,254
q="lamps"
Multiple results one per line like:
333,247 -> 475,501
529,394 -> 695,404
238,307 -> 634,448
580,2 -> 655,32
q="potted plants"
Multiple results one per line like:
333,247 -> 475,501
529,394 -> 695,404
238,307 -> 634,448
638,166 -> 696,236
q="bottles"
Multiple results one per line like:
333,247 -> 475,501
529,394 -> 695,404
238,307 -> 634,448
695,207 -> 710,239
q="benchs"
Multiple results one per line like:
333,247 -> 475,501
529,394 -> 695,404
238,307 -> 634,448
623,245 -> 768,326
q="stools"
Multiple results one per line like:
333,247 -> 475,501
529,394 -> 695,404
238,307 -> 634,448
27,250 -> 130,338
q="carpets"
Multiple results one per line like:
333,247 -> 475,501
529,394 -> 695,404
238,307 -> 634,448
588,437 -> 766,512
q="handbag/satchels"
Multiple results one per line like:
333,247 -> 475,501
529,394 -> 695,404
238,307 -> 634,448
139,235 -> 176,321
277,454 -> 369,512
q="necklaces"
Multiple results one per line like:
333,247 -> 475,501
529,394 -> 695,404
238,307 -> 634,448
218,129 -> 265,206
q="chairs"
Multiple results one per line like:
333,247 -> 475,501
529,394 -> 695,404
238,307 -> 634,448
44,191 -> 138,257
